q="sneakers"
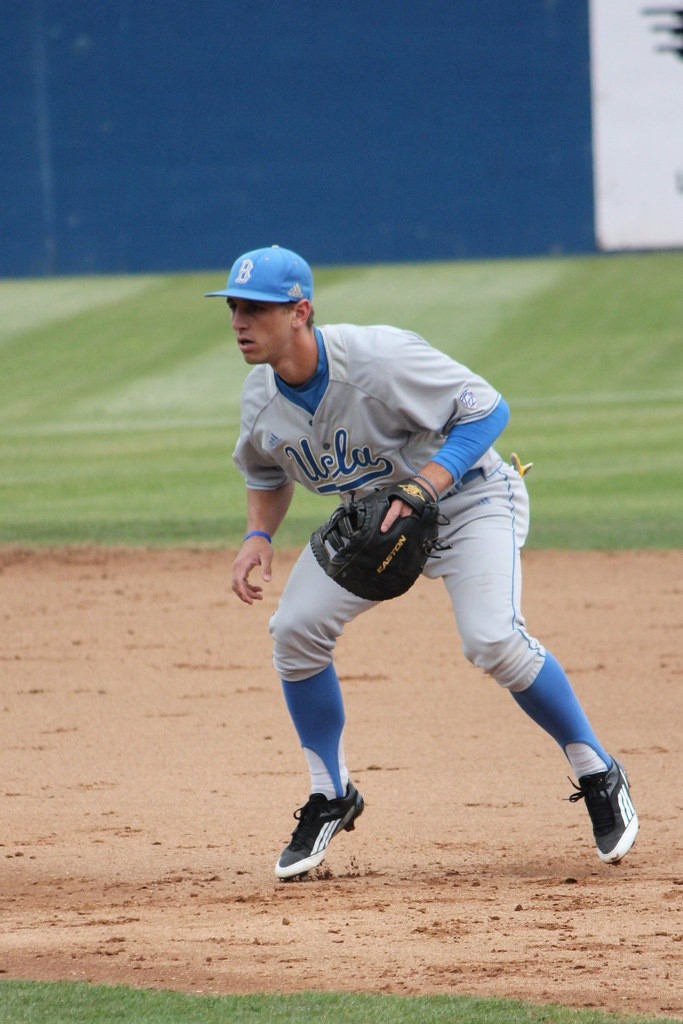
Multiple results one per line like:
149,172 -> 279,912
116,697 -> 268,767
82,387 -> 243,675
567,754 -> 638,864
275,778 -> 364,882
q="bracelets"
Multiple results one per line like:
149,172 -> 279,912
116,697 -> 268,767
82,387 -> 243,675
412,472 -> 439,501
242,530 -> 270,543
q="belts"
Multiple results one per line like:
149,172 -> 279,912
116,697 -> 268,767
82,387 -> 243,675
440,467 -> 483,500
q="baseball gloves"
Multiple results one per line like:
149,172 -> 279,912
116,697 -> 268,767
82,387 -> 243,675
308,480 -> 439,602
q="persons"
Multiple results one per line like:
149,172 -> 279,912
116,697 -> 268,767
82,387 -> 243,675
204,244 -> 640,878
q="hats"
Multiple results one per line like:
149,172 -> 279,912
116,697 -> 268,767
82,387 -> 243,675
204,245 -> 313,303
